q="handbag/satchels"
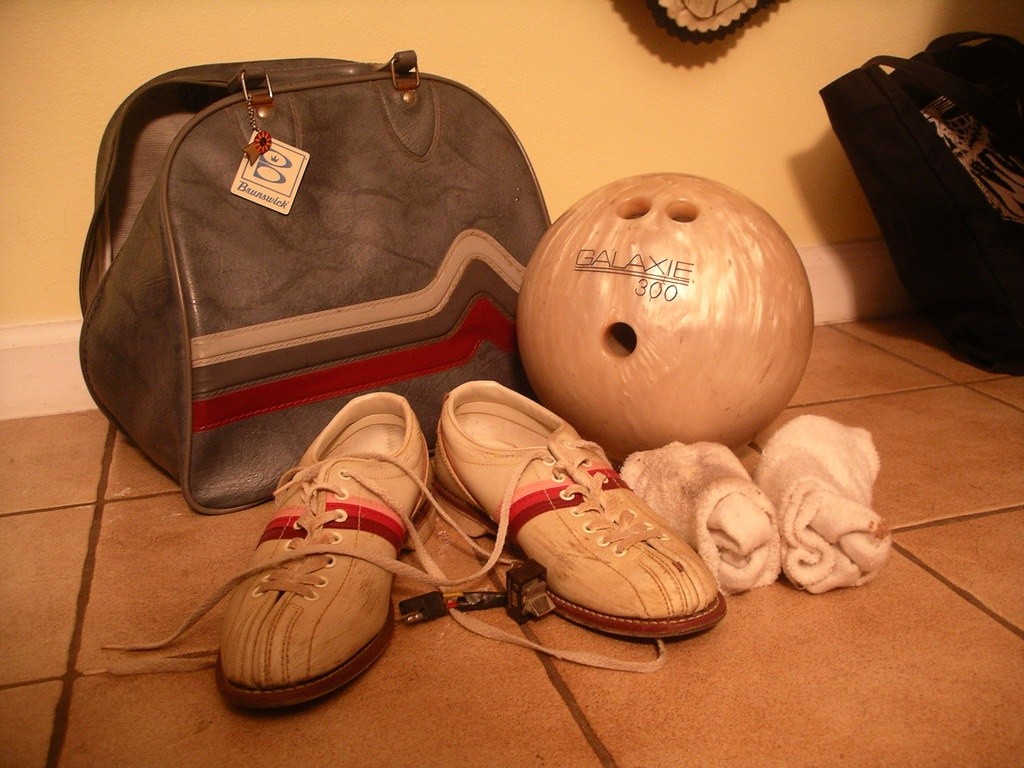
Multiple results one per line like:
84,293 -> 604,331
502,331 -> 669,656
78,49 -> 551,515
817,31 -> 1024,376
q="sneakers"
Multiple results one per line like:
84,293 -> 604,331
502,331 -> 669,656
434,380 -> 727,673
102,393 -> 432,710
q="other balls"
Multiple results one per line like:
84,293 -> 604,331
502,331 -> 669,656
512,174 -> 814,460
